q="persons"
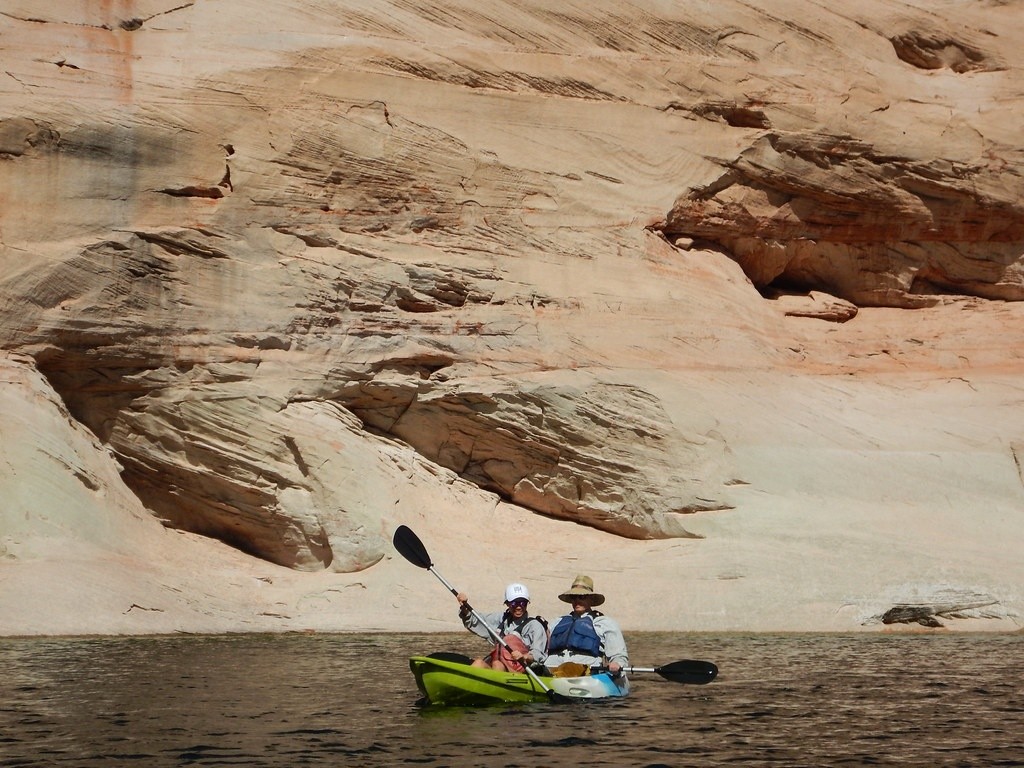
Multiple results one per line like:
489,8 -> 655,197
457,582 -> 548,674
540,574 -> 629,679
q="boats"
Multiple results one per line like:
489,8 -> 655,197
407,649 -> 631,709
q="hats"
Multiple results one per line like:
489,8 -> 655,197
558,575 -> 605,607
503,583 -> 530,603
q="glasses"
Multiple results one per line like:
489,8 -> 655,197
506,600 -> 527,609
571,594 -> 590,601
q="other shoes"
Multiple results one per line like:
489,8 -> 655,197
531,663 -> 553,677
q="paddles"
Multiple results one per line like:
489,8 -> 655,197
538,659 -> 719,685
393,524 -> 576,705
